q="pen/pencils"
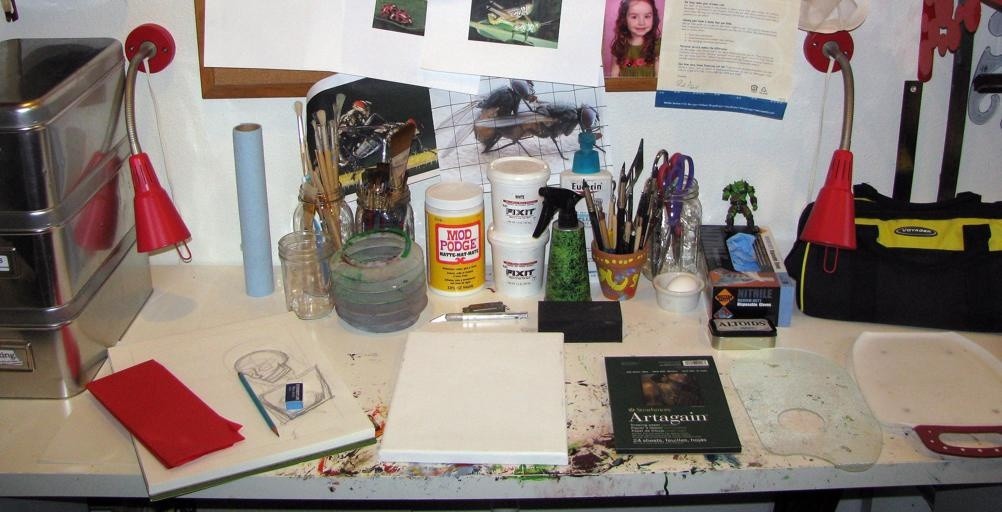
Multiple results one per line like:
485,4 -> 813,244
429,313 -> 528,324
238,372 -> 279,437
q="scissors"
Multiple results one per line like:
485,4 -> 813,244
652,149 -> 694,277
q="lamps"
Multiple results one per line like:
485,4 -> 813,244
121,22 -> 193,254
799,31 -> 857,253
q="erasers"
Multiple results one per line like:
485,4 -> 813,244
285,383 -> 303,410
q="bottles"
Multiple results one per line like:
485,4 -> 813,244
642,176 -> 703,282
486,222 -> 549,301
354,181 -> 417,242
425,180 -> 487,297
294,181 -> 355,250
331,227 -> 429,332
486,155 -> 550,236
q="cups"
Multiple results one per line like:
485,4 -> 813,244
590,239 -> 705,311
277,231 -> 336,320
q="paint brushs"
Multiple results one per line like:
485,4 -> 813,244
294,94 -> 416,291
582,164 -> 662,253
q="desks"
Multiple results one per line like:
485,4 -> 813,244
1,262 -> 1002,511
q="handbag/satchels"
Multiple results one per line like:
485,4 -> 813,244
784,182 -> 1002,334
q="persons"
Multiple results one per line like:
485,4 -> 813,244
609,0 -> 661,79
